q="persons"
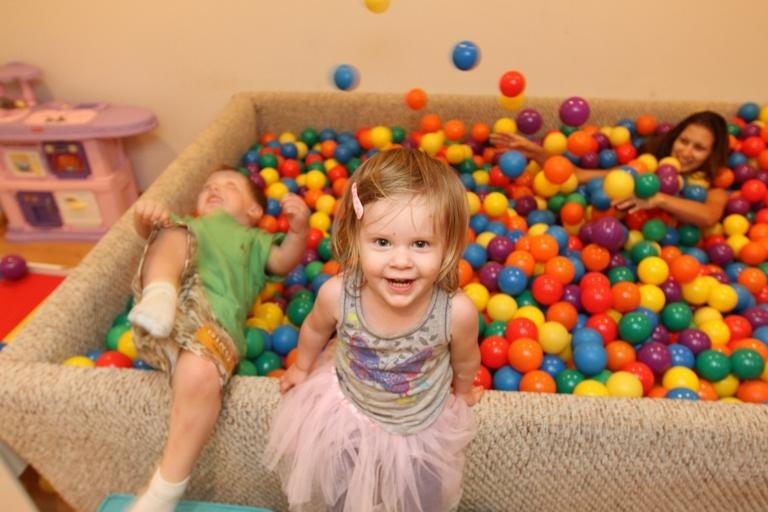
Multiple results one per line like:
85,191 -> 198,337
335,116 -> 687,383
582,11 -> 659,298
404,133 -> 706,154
490,111 -> 727,226
260,146 -> 485,512
123,164 -> 310,512
